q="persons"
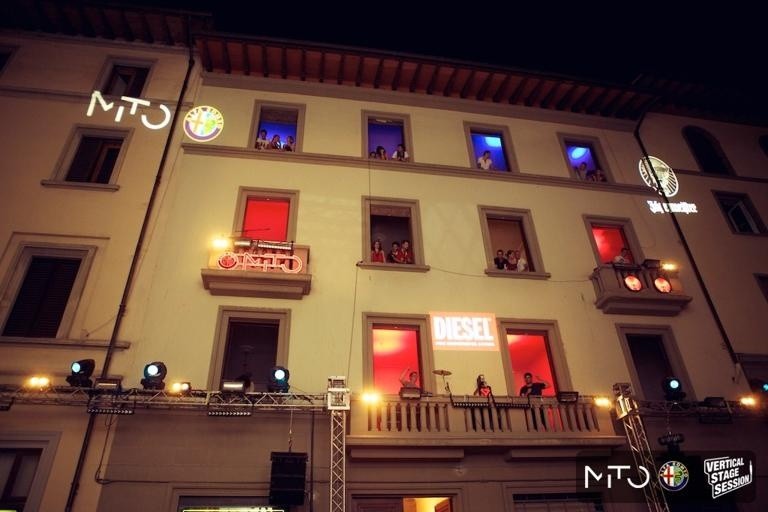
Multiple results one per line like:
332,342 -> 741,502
386,242 -> 403,263
375,146 -> 388,160
283,135 -> 294,152
400,239 -> 411,263
624,249 -> 633,263
519,372 -> 551,397
504,241 -> 524,270
477,150 -> 495,170
391,143 -> 409,161
574,161 -> 606,182
614,248 -> 630,264
514,251 -> 529,272
256,129 -> 269,150
270,134 -> 281,149
494,250 -> 507,269
398,364 -> 419,387
474,374 -> 493,397
370,151 -> 376,159
371,240 -> 386,264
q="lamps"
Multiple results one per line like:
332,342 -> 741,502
65,358 -> 290,393
399,376 -> 686,404
